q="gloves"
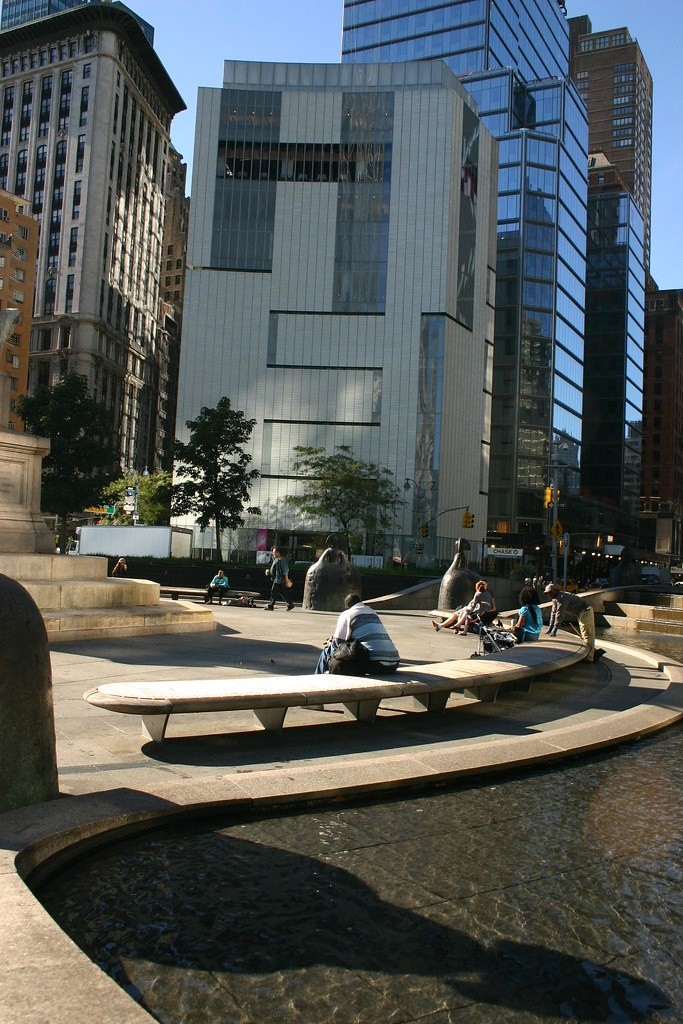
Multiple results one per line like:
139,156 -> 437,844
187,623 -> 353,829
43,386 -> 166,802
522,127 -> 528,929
551,626 -> 557,637
545,625 -> 553,635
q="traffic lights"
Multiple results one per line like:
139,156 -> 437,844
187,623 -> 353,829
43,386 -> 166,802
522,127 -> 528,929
462,513 -> 477,530
420,526 -> 435,541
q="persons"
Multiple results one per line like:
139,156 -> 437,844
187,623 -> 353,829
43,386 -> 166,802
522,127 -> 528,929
111,557 -> 127,578
263,547 -> 296,611
299,593 -> 401,712
543,583 -> 595,662
512,586 -> 542,644
205,570 -> 229,606
432,578 -> 496,636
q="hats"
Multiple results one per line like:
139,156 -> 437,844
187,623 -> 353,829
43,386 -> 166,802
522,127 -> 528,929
118,558 -> 125,562
543,584 -> 555,594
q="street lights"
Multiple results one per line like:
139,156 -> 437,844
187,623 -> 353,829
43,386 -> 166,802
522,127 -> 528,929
481,536 -> 495,577
545,434 -> 568,581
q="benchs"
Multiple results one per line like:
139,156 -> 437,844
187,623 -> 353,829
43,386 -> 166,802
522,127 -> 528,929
159,586 -> 262,604
84,609 -> 590,743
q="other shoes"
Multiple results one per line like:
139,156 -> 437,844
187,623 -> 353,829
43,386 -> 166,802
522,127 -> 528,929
454,630 -> 458,634
450,626 -> 460,629
576,659 -> 592,664
458,632 -> 467,635
286,604 -> 295,611
206,601 -> 212,605
298,704 -> 324,710
264,606 -> 273,611
432,621 -> 440,632
218,602 -> 222,605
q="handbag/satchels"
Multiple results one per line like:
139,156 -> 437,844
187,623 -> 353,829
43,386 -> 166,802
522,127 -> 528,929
328,639 -> 370,675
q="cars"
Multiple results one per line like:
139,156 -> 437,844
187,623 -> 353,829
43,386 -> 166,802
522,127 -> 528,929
546,565 -> 683,595
